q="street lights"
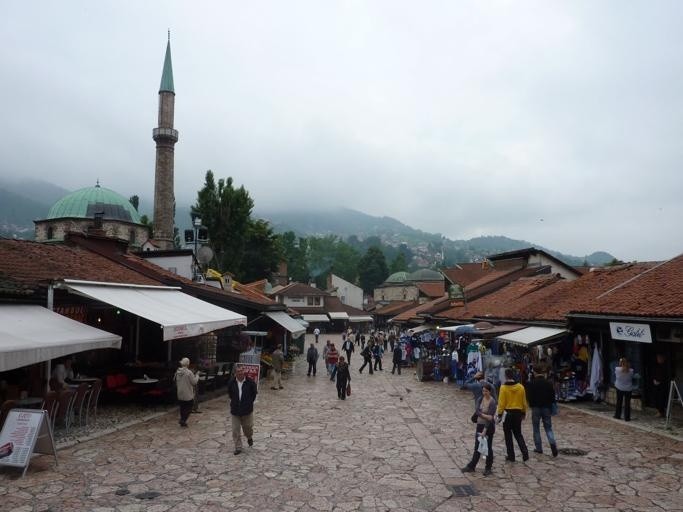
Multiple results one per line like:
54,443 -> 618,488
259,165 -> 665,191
184,217 -> 208,285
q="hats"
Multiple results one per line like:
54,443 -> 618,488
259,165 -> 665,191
180,357 -> 190,366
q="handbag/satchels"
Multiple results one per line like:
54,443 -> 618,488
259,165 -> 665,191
347,383 -> 351,396
472,412 -> 478,423
551,402 -> 558,416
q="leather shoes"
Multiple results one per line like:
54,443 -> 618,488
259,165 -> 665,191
484,469 -> 490,475
462,465 -> 472,472
248,439 -> 253,446
234,450 -> 241,454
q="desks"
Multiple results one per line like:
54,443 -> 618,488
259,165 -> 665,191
132,379 -> 159,409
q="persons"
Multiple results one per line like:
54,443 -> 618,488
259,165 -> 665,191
330,356 -> 351,398
227,363 -> 257,456
652,353 -> 669,418
459,366 -> 560,476
48,359 -> 74,391
611,358 -> 633,422
270,344 -> 284,391
304,327 -> 403,378
124,358 -> 160,384
173,357 -> 201,427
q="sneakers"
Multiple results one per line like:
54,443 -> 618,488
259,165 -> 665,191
534,448 -> 542,453
505,456 -> 515,461
550,444 -> 557,457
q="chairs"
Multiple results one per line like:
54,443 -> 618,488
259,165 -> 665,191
0,378 -> 102,436
